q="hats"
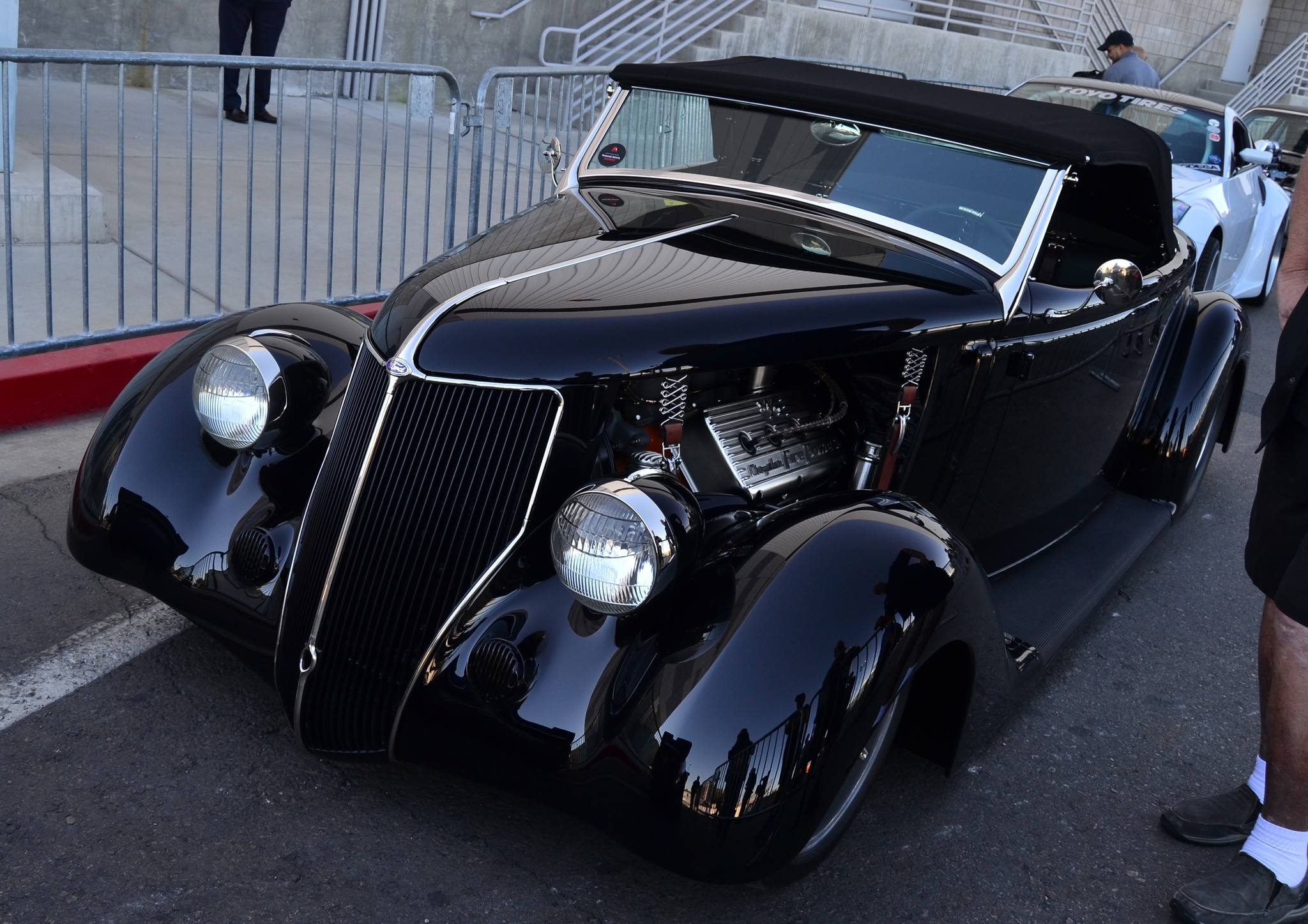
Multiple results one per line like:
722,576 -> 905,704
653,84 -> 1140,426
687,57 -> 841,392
1096,28 -> 1135,52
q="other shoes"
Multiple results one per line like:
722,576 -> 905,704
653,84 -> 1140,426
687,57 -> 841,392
1169,845 -> 1308,924
1160,772 -> 1268,844
248,108 -> 279,124
225,104 -> 250,123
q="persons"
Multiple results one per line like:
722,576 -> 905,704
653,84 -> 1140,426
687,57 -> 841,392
1095,27 -> 1159,93
219,0 -> 292,125
1160,139 -> 1308,924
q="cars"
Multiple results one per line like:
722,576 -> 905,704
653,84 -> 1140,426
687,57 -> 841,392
66,54 -> 1250,886
1242,106 -> 1308,201
1003,75 -> 1292,312
